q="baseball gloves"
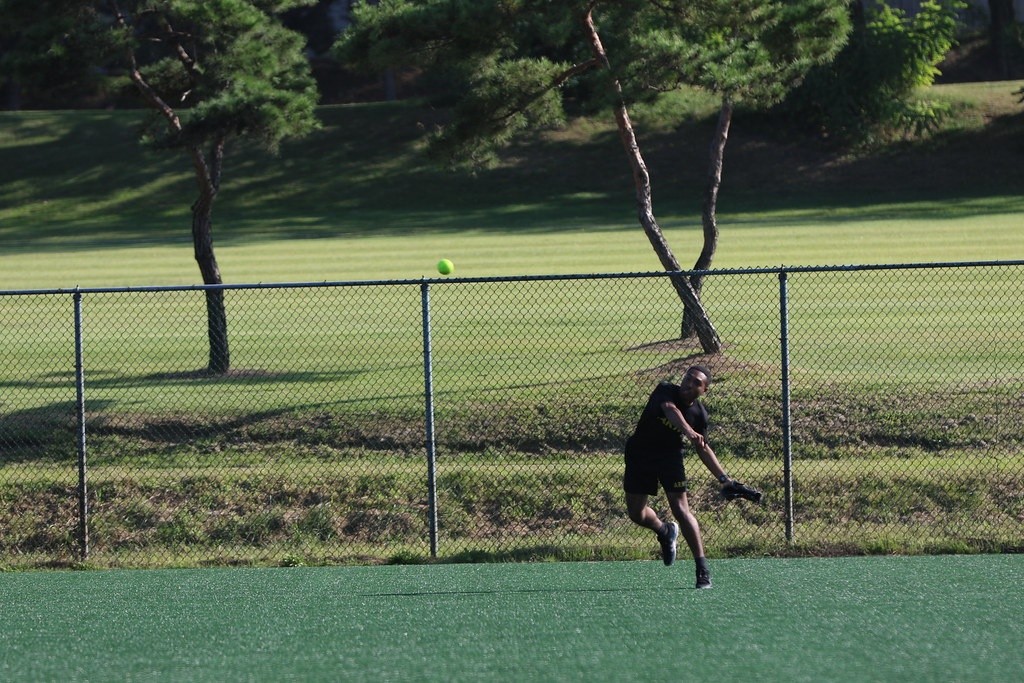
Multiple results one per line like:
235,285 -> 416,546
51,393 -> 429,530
721,480 -> 762,504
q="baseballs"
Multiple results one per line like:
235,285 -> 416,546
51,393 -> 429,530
438,258 -> 454,275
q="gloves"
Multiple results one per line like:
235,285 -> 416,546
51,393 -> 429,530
718,474 -> 744,500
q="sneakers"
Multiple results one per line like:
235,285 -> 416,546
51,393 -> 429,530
657,522 -> 679,566
696,568 -> 711,589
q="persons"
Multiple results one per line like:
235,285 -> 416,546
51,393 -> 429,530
623,365 -> 732,590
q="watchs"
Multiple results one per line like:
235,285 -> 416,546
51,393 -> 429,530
720,475 -> 728,483
